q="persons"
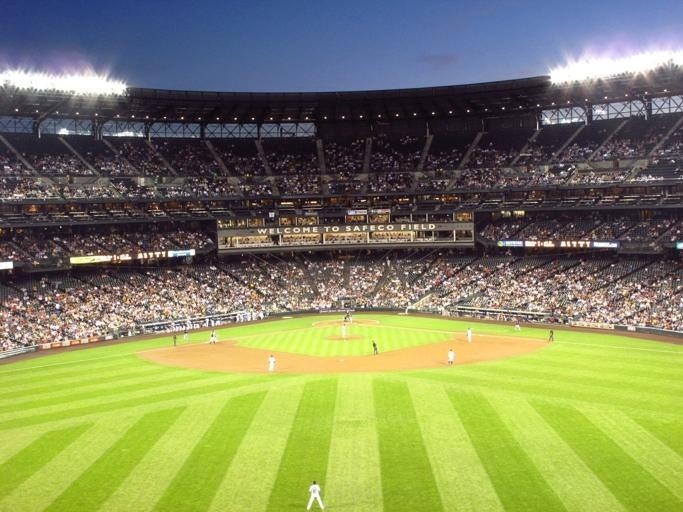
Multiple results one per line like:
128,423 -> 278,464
306,481 -> 323,510
268,355 -> 274,371
0,122 -> 683,346
372,341 -> 378,356
448,350 -> 453,366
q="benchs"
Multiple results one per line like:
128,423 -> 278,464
259,248 -> 436,290
0,260 -> 247,306
445,251 -> 682,291
637,161 -> 680,178
